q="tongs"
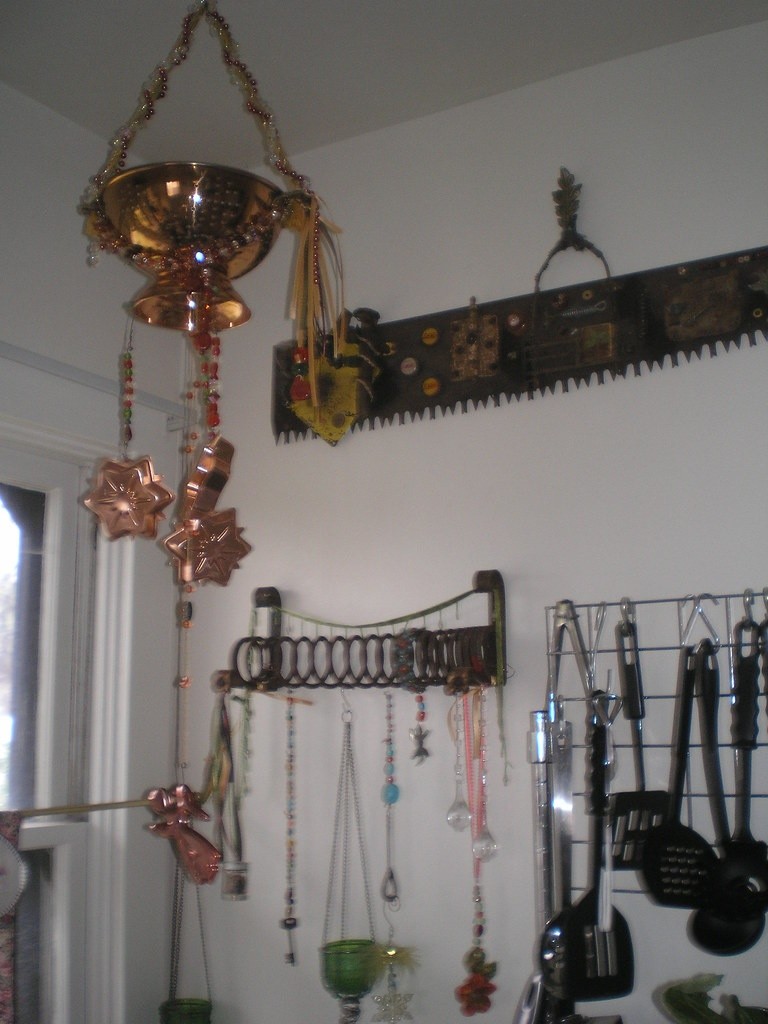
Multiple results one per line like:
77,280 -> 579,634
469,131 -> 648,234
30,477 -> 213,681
543,600 -> 620,781
